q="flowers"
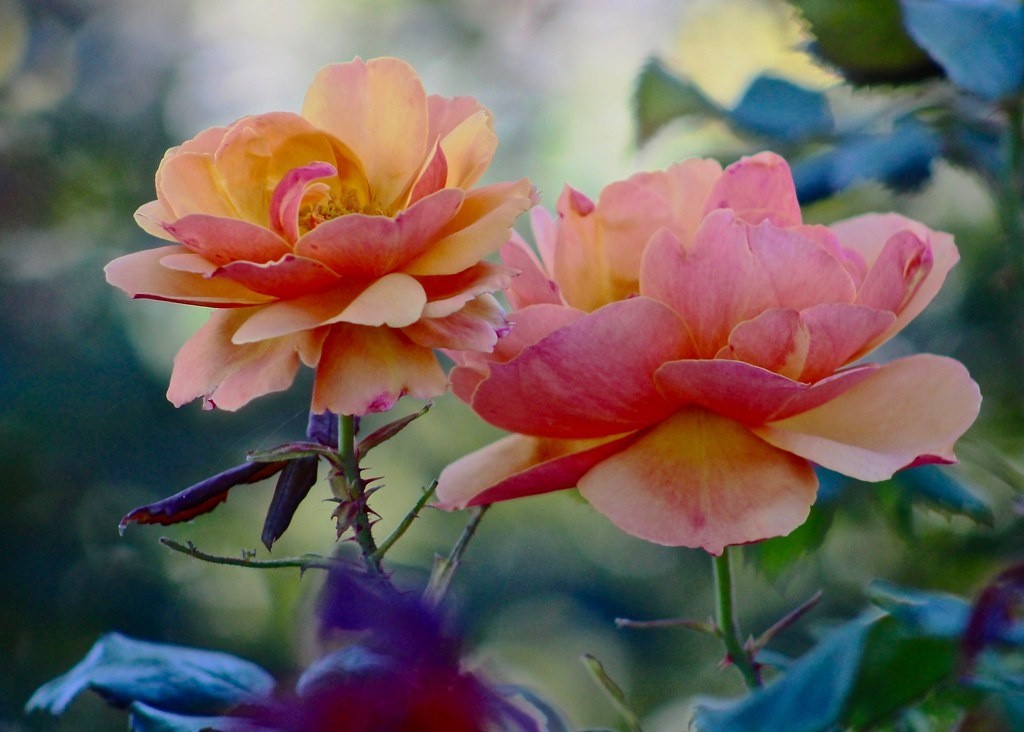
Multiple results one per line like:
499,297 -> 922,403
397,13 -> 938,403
24,54 -> 1024,732
